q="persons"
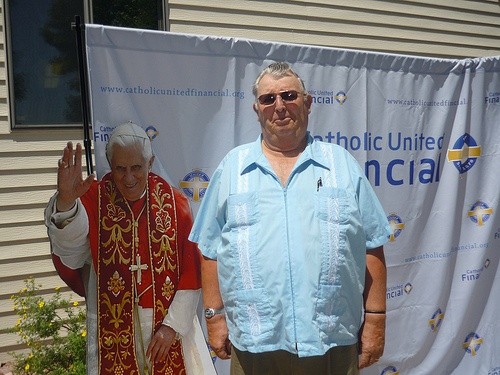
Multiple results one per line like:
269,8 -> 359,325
189,62 -> 393,375
45,122 -> 219,375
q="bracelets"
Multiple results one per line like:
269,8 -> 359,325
365,311 -> 385,313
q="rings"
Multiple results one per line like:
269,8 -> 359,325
64,165 -> 69,168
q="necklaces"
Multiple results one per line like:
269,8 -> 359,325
129,203 -> 148,285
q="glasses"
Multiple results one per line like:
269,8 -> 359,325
257,90 -> 307,104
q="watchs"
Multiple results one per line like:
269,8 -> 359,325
204,307 -> 224,319
